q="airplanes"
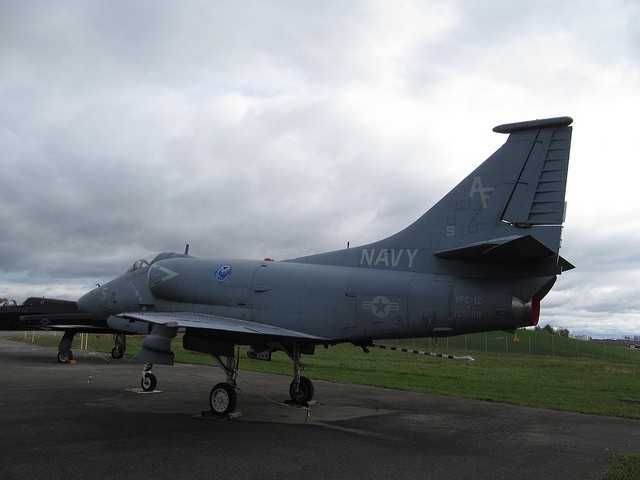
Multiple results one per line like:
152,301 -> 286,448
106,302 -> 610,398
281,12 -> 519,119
76,116 -> 574,420
0,297 -> 137,364
